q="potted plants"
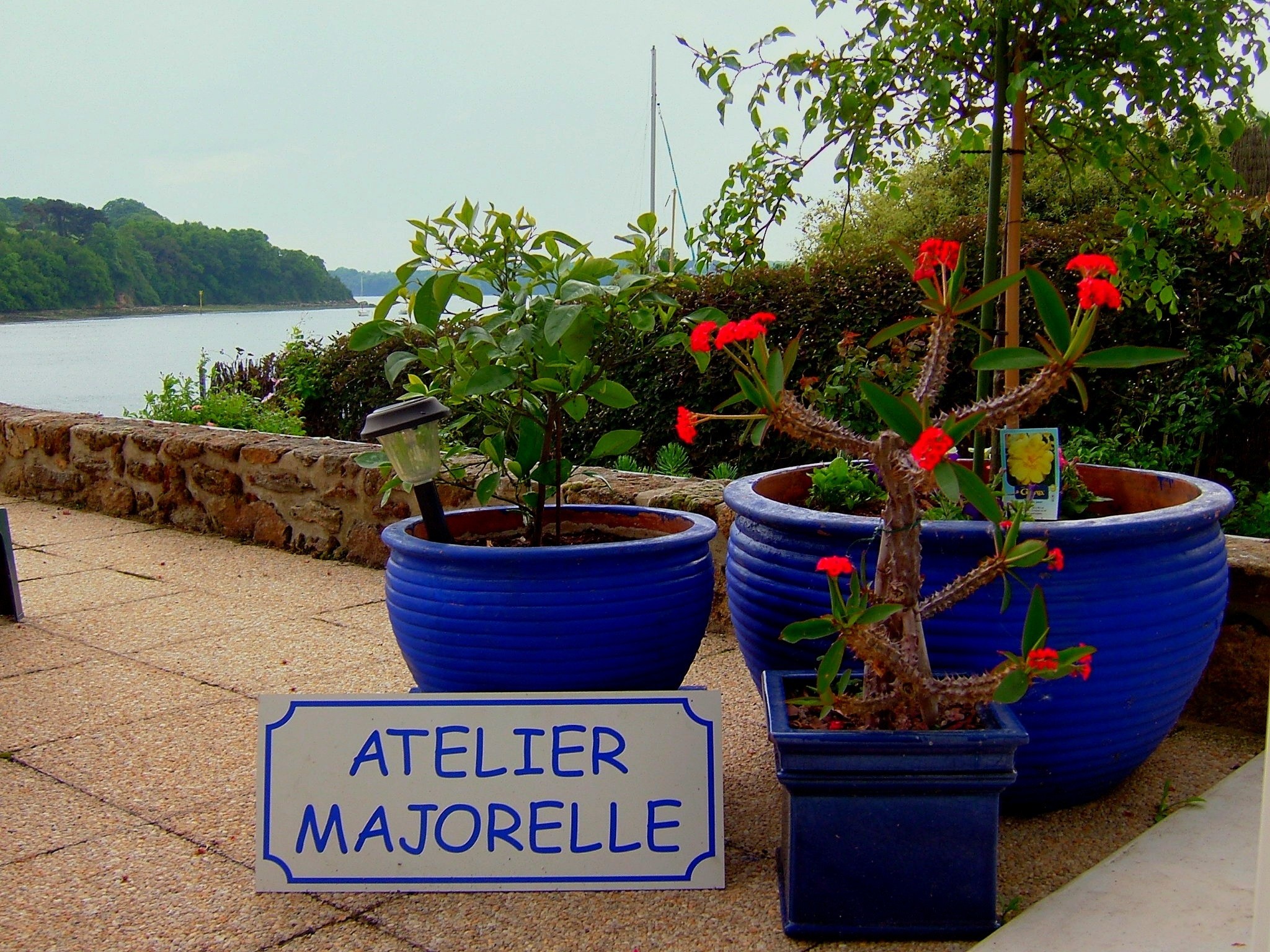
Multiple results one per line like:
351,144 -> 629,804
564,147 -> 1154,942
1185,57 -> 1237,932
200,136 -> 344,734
347,196 -> 730,693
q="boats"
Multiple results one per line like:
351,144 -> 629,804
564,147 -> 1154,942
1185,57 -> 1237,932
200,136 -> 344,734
399,307 -> 408,315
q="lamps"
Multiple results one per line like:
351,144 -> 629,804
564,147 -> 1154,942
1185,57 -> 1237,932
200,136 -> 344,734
362,396 -> 461,545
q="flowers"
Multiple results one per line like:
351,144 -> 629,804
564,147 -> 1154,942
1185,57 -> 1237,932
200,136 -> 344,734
802,449 -> 1114,522
675,239 -> 1190,730
675,0 -> 1270,525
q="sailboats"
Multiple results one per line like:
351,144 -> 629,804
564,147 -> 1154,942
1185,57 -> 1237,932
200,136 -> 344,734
356,276 -> 370,317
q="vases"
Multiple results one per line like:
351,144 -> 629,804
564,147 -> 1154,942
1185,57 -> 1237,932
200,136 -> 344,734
763,671 -> 1029,942
726,458 -> 1233,815
